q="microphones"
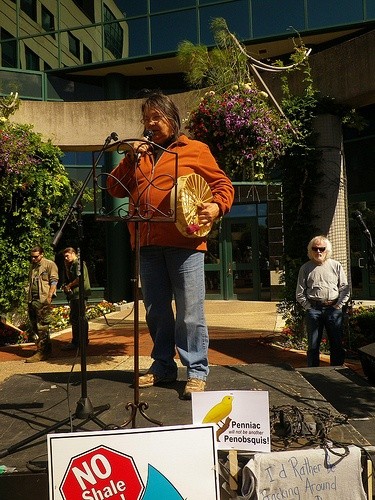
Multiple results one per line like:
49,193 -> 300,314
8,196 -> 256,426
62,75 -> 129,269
137,129 -> 153,159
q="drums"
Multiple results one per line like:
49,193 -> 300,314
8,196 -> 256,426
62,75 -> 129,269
169,174 -> 215,238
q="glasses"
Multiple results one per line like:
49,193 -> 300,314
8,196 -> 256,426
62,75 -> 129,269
31,254 -> 40,258
312,247 -> 326,251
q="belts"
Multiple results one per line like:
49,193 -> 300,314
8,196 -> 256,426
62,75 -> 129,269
308,299 -> 337,306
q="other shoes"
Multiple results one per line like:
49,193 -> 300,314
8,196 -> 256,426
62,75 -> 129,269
66,343 -> 89,350
26,351 -> 52,363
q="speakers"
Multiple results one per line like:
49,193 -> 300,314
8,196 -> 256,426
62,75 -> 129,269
357,342 -> 375,387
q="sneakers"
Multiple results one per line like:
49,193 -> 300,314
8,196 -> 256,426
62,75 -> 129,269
183,378 -> 207,400
139,371 -> 176,387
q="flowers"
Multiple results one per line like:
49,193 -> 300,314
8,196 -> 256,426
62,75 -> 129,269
187,91 -> 302,180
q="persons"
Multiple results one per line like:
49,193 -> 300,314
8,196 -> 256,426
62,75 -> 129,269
61,247 -> 91,352
296,235 -> 350,368
107,93 -> 234,399
26,245 -> 59,363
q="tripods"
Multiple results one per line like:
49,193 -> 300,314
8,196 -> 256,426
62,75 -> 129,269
0,140 -> 163,459
